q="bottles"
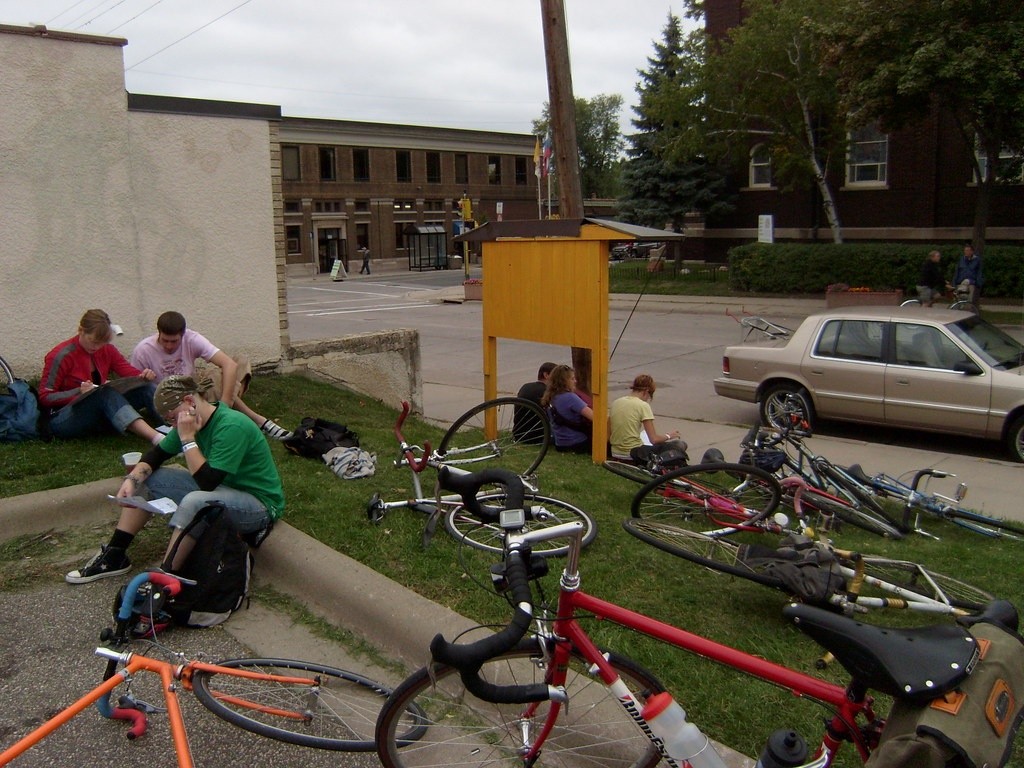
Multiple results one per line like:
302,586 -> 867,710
639,687 -> 728,767
754,729 -> 808,768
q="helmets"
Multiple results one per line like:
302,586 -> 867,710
112,578 -> 174,640
738,447 -> 788,472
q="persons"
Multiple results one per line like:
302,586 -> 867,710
921,244 -> 984,308
359,239 -> 371,274
607,374 -> 681,465
38,309 -> 294,447
512,362 -> 612,457
64,374 -> 286,596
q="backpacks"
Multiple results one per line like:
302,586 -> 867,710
284,418 -> 359,459
162,500 -> 255,628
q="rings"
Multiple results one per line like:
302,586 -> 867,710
186,413 -> 189,415
190,413 -> 196,416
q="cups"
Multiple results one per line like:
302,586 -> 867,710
122,451 -> 143,474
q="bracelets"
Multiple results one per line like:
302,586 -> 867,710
182,441 -> 198,453
123,475 -> 141,492
664,433 -> 670,440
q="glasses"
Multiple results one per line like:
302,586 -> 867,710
166,419 -> 177,425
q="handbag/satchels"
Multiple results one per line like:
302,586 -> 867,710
744,533 -> 848,605
866,617 -> 1024,768
0,357 -> 41,439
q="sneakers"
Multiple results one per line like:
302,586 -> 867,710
65,542 -> 132,584
259,419 -> 293,441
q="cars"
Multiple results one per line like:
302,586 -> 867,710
612,241 -> 665,260
711,307 -> 1023,465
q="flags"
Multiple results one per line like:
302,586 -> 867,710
533,136 -> 552,180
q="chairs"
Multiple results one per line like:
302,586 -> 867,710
911,331 -> 942,367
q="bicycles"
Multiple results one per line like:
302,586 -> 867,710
900,286 -> 980,330
0,356 -> 14,390
367,397 -> 596,557
0,569 -> 428,768
598,387 -> 1023,617
378,473 -> 981,768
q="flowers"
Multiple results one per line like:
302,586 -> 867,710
462,278 -> 483,285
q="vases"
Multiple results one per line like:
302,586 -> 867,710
465,285 -> 483,300
